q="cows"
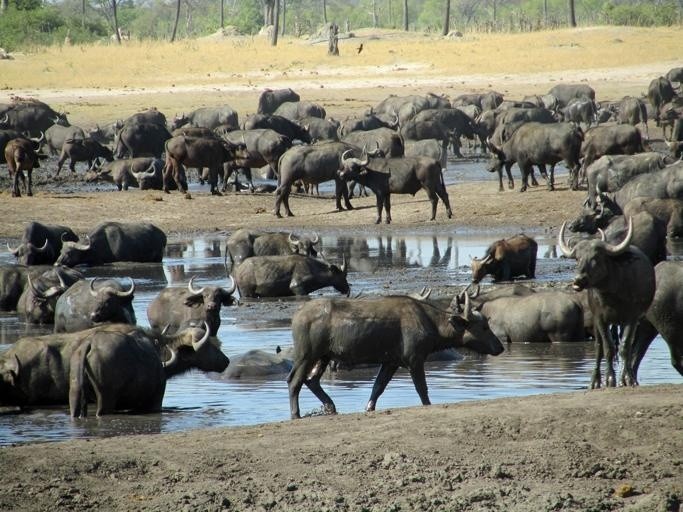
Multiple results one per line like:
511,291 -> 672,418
0,67 -> 683,223
0,211 -> 682,419
